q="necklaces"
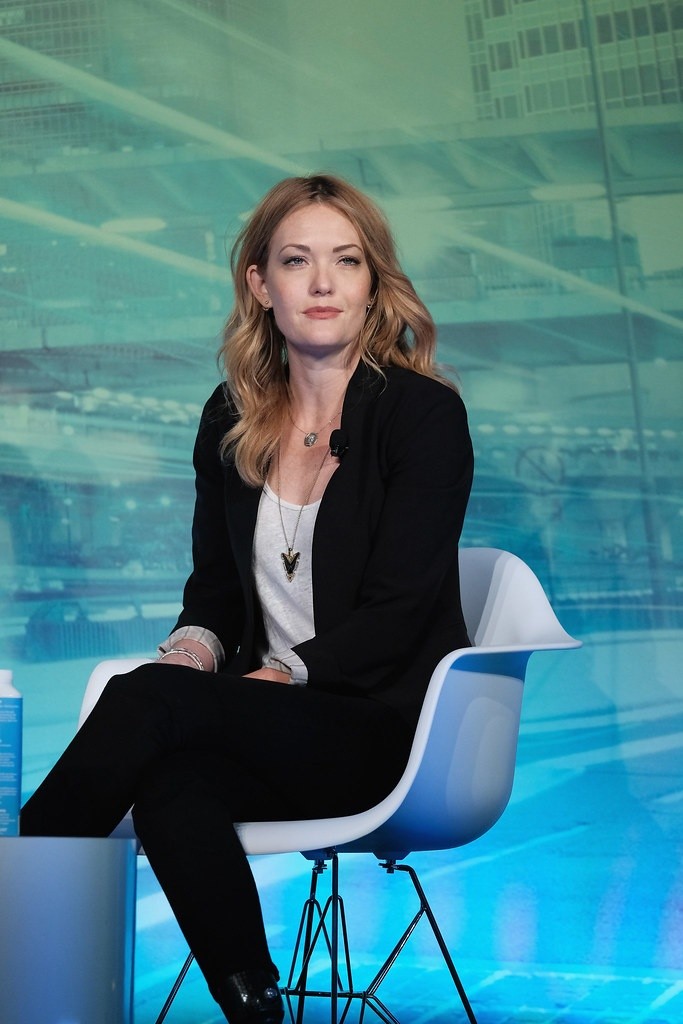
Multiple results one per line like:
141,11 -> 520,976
287,408 -> 342,447
276,440 -> 331,582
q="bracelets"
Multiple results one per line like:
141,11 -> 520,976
160,647 -> 204,671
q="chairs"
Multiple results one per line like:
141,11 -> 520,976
80,543 -> 584,1024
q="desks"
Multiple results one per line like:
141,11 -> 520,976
0,837 -> 135,1024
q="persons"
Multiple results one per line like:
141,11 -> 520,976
18,173 -> 474,1024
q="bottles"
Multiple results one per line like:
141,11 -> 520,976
0,668 -> 23,838
